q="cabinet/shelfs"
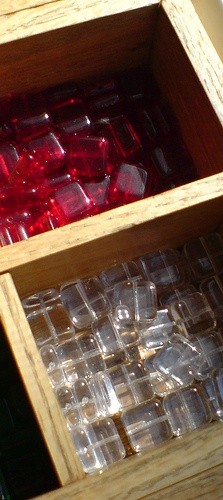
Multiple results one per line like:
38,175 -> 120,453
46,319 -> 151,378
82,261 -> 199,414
0,0 -> 223,500
